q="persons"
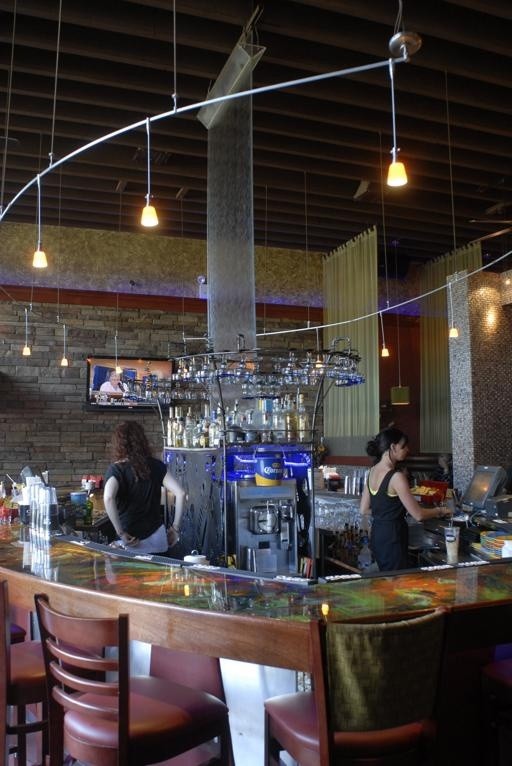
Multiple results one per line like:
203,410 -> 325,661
358,426 -> 454,571
101,420 -> 187,557
99,368 -> 130,392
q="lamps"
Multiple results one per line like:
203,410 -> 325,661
0,0 -> 511,370
391,239 -> 411,406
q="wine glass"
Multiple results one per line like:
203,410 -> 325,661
156,348 -> 366,406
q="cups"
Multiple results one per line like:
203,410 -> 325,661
444,526 -> 460,565
344,469 -> 361,496
0,482 -> 62,535
19,527 -> 63,582
69,490 -> 87,520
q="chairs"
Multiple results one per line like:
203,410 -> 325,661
0,575 -> 104,766
481,660 -> 512,766
10,622 -> 26,766
263,605 -> 448,766
34,594 -> 229,766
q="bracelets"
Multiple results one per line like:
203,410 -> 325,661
118,529 -> 126,538
433,506 -> 444,519
171,524 -> 180,534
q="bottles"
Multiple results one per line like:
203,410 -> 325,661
331,522 -> 373,570
166,393 -> 311,449
306,468 -> 325,491
